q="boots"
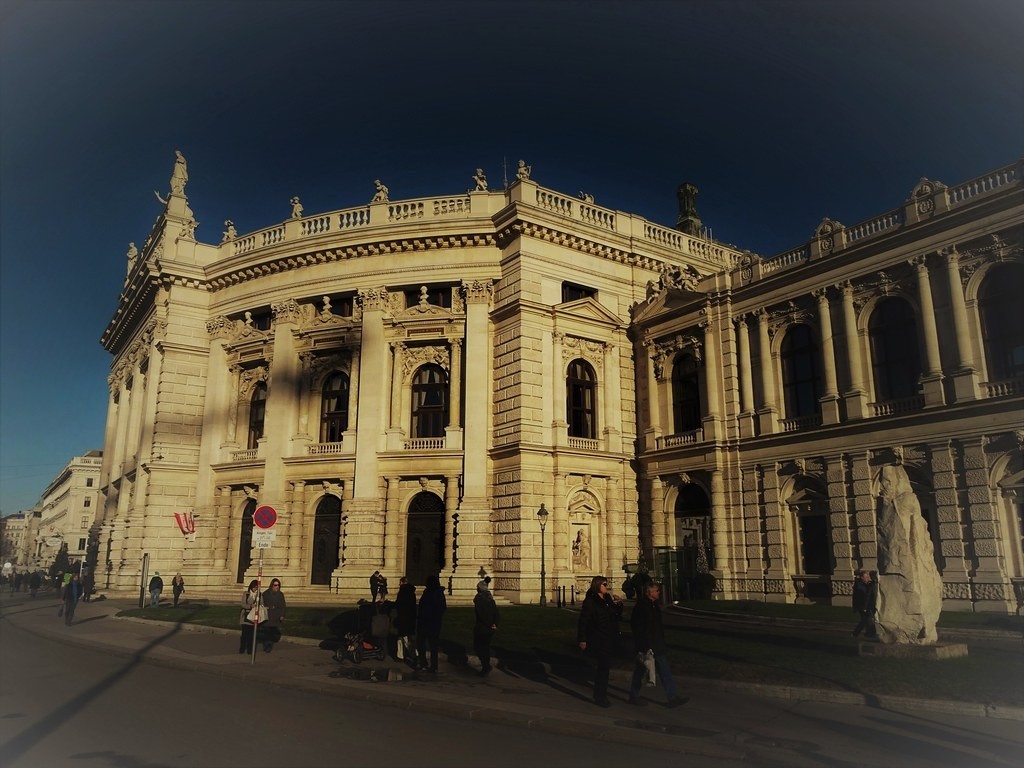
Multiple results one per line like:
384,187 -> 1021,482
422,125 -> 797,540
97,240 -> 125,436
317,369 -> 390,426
481,656 -> 493,677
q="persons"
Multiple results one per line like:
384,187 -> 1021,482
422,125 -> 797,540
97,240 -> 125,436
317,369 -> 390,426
154,190 -> 169,205
239,580 -> 264,658
127,242 -> 138,275
0,571 -> 95,626
579,576 -> 624,705
472,169 -> 488,192
290,197 -> 303,218
371,180 -> 388,202
515,160 -> 531,181
172,573 -> 184,608
222,220 -> 237,241
628,583 -> 691,710
346,571 -> 446,673
851,571 -> 879,638
149,572 -> 163,608
261,578 -> 286,658
473,577 -> 499,674
170,151 -> 188,194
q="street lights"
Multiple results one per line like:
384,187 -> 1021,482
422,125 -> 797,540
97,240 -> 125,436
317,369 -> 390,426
537,503 -> 550,609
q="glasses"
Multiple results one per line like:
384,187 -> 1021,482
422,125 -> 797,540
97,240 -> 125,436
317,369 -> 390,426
271,583 -> 281,587
602,583 -> 608,587
653,590 -> 660,593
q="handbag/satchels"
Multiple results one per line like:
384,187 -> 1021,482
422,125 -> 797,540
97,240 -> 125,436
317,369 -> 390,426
642,649 -> 657,688
90,586 -> 97,595
58,600 -> 65,617
397,635 -> 409,659
243,612 -> 256,624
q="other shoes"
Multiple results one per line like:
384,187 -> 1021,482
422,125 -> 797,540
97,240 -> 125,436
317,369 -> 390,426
83,599 -> 85,602
431,666 -> 438,672
64,623 -> 73,626
422,665 -> 429,671
239,644 -> 245,654
406,655 -> 414,663
595,698 -> 611,707
247,648 -> 255,655
594,691 -> 598,697
87,599 -> 90,603
629,695 -> 651,705
667,688 -> 689,708
263,645 -> 271,653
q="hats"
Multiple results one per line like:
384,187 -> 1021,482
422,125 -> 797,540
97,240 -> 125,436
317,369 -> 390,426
154,571 -> 160,576
477,576 -> 491,591
